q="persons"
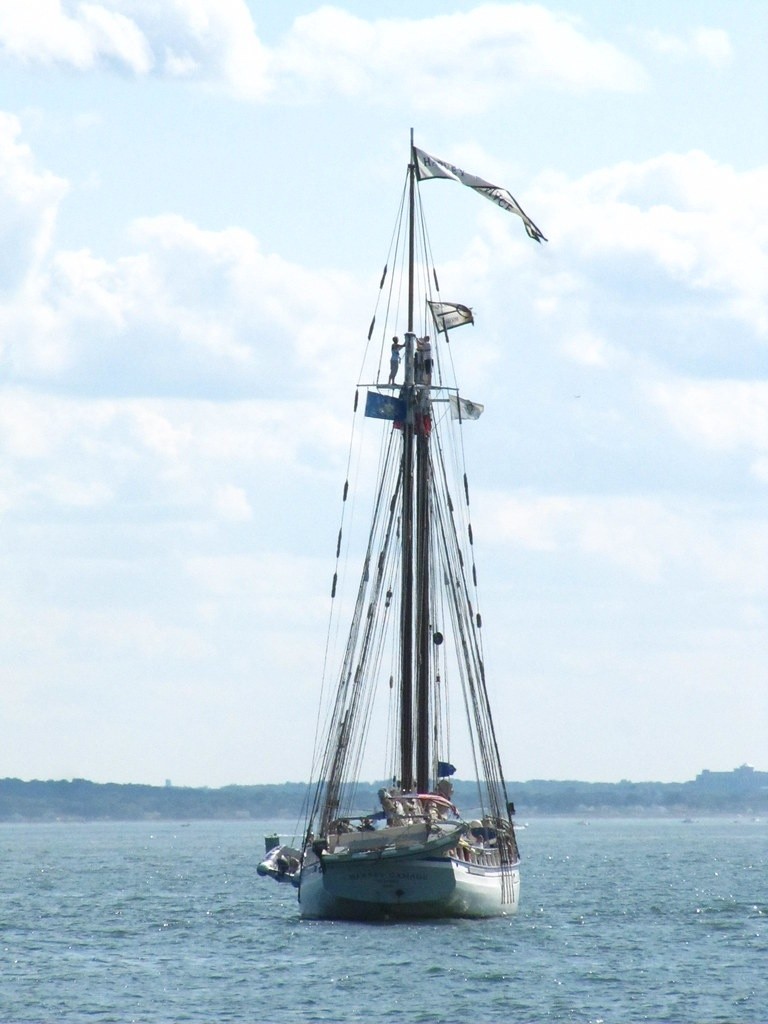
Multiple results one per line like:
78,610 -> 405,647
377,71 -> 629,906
472,834 -> 485,848
388,336 -> 405,385
412,334 -> 434,385
361,818 -> 375,832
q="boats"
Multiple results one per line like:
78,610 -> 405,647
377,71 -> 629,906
322,819 -> 470,863
256,843 -> 305,886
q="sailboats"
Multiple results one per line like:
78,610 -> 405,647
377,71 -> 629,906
292,125 -> 550,920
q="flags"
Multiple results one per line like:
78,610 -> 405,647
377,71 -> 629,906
414,145 -> 548,243
364,389 -> 409,423
429,298 -> 475,335
449,393 -> 482,423
391,412 -> 434,438
